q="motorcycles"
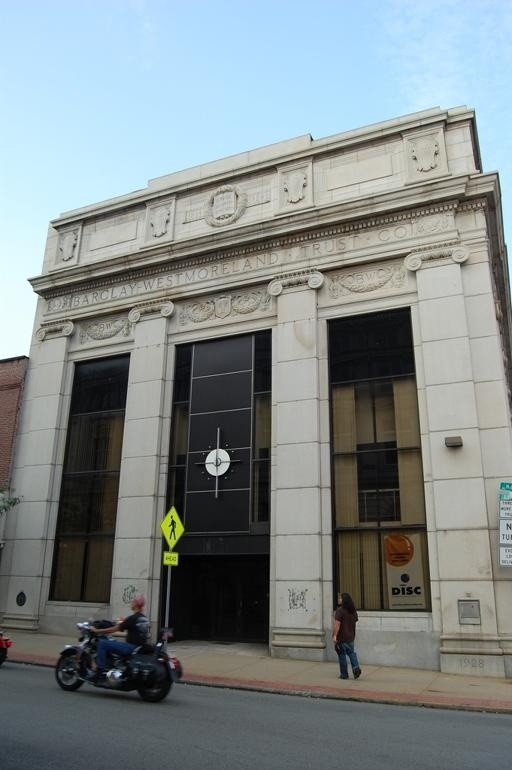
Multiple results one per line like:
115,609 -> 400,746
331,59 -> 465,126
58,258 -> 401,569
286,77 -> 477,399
54,604 -> 182,703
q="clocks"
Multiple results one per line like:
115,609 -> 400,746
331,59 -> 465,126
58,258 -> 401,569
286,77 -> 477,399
192,427 -> 241,500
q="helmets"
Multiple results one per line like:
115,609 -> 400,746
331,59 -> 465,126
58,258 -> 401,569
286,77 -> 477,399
131,595 -> 146,608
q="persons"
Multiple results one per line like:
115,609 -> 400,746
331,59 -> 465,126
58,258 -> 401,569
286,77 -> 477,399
88,595 -> 151,676
332,592 -> 362,682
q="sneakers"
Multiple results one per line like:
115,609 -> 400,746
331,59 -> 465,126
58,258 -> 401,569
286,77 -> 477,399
353,665 -> 362,680
83,670 -> 107,681
335,673 -> 350,679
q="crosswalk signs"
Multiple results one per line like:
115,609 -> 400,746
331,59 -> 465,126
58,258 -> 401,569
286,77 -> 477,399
160,506 -> 185,550
